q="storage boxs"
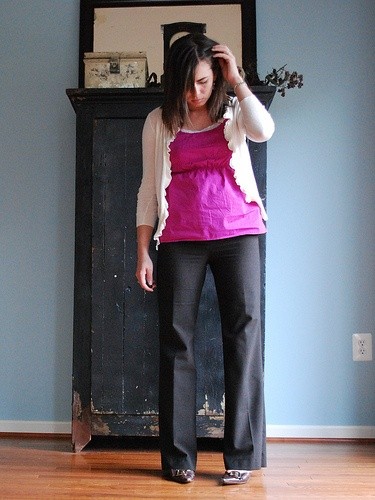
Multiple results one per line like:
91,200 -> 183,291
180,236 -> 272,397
84,52 -> 148,89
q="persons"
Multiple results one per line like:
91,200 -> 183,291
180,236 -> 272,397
135,32 -> 275,484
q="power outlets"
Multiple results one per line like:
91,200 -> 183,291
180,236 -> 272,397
353,334 -> 372,361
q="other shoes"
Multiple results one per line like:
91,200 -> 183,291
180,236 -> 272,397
166,468 -> 194,484
219,468 -> 254,484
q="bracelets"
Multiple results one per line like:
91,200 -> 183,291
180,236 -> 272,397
233,82 -> 246,94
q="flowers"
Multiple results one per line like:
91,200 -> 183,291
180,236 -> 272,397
263,63 -> 302,97
